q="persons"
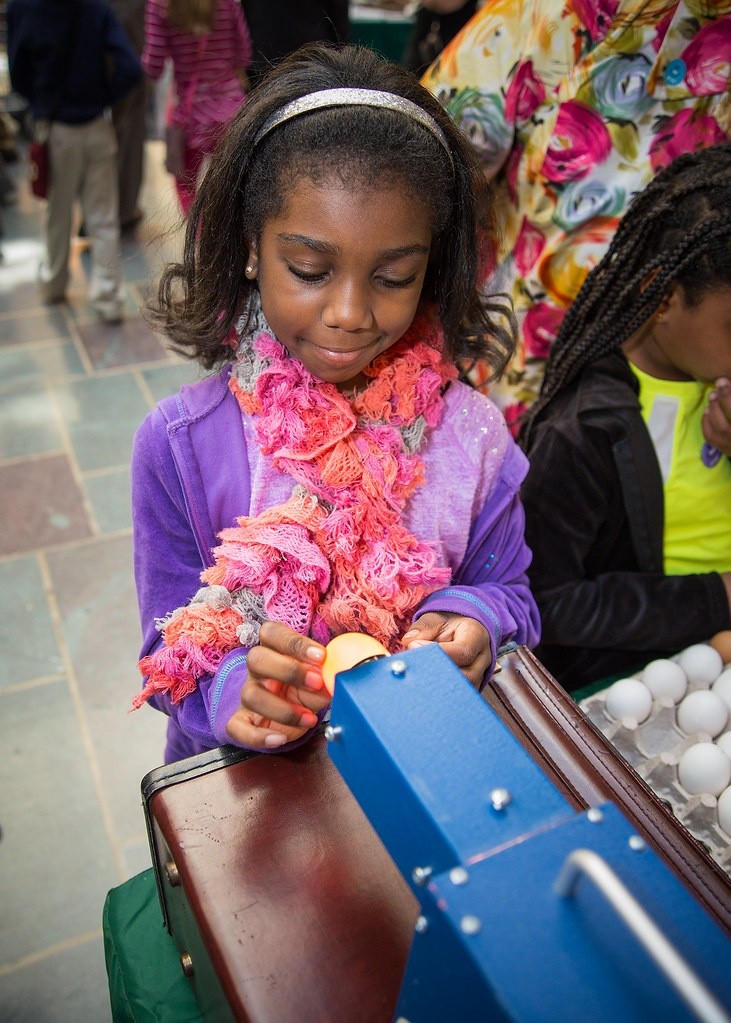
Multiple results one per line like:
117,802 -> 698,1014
6,0 -> 257,326
514,144 -> 731,682
131,41 -> 540,765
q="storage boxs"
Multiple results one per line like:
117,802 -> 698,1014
136,639 -> 731,1023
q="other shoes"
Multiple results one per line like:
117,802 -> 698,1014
97,309 -> 122,326
39,288 -> 65,304
117,206 -> 144,234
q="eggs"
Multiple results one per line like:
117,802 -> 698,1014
605,629 -> 731,838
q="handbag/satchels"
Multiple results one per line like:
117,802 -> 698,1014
27,126 -> 51,200
